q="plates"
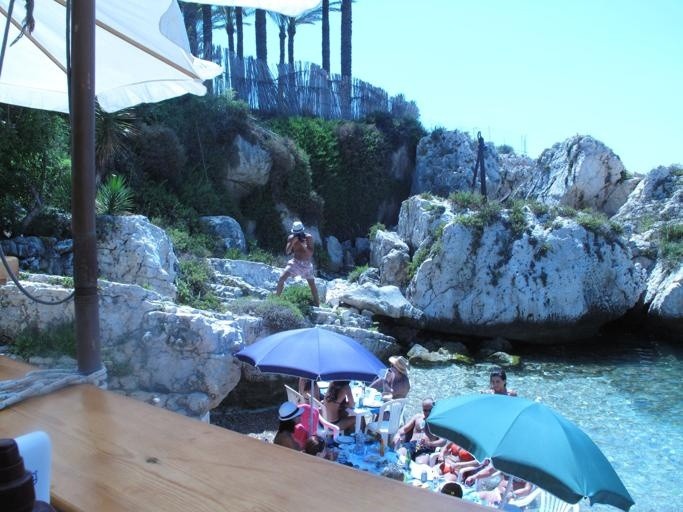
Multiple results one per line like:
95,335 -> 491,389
337,436 -> 355,443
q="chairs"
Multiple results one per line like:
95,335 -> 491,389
306,392 -> 345,438
385,432 -> 579,512
282,384 -> 305,425
366,397 -> 404,439
317,416 -> 355,445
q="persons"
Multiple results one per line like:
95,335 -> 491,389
394,398 -> 532,510
274,355 -> 410,461
486,369 -> 517,396
277,220 -> 322,309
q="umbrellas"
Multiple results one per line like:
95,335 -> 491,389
423,388 -> 636,512
228,321 -> 390,439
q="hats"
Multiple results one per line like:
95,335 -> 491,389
389,355 -> 408,374
290,221 -> 304,234
278,401 -> 305,421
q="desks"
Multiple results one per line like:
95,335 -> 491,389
320,384 -> 393,434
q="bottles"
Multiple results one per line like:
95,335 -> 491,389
355,429 -> 364,453
405,449 -> 411,470
358,389 -> 364,408
380,439 -> 385,457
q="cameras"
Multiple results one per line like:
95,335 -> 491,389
295,232 -> 305,243
